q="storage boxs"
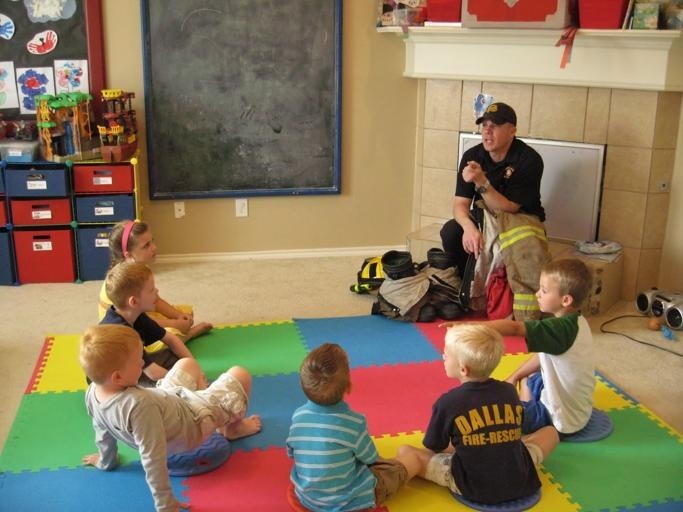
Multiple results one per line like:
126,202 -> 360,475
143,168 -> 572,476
664,6 -> 683,30
461,0 -> 576,29
576,0 -> 626,29
425,0 -> 461,23
0,159 -> 140,287
633,3 -> 672,30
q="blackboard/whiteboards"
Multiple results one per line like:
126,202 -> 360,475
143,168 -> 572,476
457,133 -> 606,244
140,0 -> 343,200
0,0 -> 107,123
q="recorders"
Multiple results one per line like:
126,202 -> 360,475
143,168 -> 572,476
635,286 -> 683,330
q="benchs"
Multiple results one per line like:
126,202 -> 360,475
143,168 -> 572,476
405,220 -> 625,317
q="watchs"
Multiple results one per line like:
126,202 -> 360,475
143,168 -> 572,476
474,179 -> 490,195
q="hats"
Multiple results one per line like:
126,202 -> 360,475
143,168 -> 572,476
476,101 -> 516,125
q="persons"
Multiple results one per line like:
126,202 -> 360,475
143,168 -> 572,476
434,256 -> 598,438
80,323 -> 263,512
282,342 -> 422,512
393,324 -> 562,502
95,260 -> 208,391
98,218 -> 211,354
440,101 -> 546,279
